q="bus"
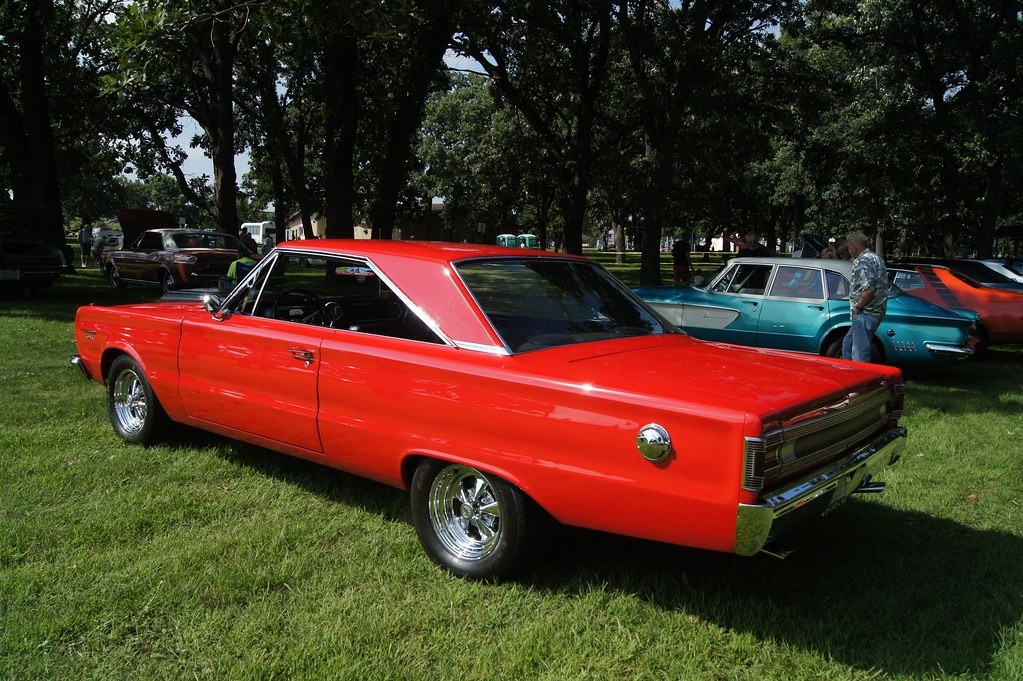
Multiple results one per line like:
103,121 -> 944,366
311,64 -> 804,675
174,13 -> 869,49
241,221 -> 277,247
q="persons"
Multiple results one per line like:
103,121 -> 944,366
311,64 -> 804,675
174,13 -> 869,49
832,234 -> 861,295
800,247 -> 837,292
841,231 -> 889,364
218,245 -> 260,300
75,223 -> 93,269
671,228 -> 695,289
692,266 -> 705,288
240,224 -> 279,258
735,225 -> 774,292
89,234 -> 106,267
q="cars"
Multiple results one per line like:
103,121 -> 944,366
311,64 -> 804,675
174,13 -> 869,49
90,233 -> 123,261
74,236 -> 907,590
880,261 -> 1023,357
907,255 -> 1023,291
628,254 -> 983,376
101,228 -> 265,302
